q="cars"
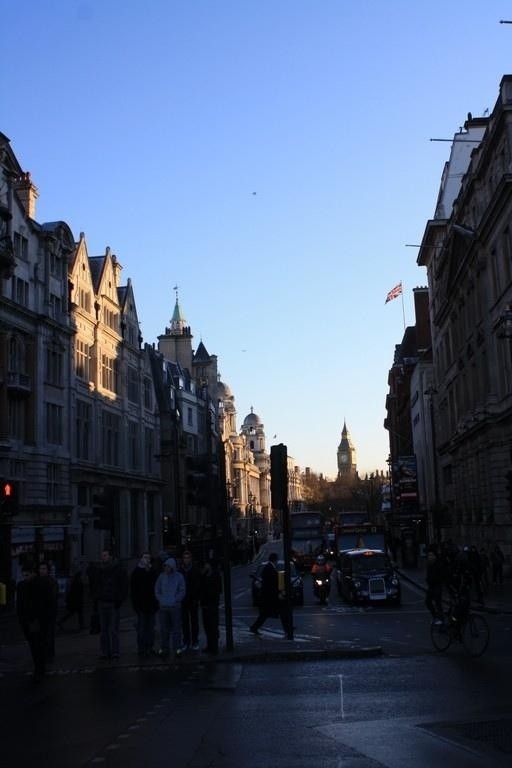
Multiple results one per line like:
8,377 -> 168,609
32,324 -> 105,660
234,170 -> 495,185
249,560 -> 304,609
334,547 -> 401,608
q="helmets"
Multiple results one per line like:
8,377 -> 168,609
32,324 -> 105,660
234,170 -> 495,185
316,555 -> 325,565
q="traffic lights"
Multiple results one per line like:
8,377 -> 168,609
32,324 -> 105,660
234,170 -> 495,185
183,452 -> 213,508
270,444 -> 289,511
92,493 -> 112,530
0,478 -> 20,517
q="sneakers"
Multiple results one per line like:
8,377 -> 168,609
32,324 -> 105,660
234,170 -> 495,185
100,641 -> 221,662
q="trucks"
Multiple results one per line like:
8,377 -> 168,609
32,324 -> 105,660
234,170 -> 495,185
333,510 -> 387,567
289,510 -> 330,568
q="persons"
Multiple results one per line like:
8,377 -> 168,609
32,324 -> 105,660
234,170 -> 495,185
193,546 -> 222,657
86,548 -> 124,660
176,548 -> 204,653
246,551 -> 298,636
74,552 -> 101,635
421,538 -> 505,623
128,551 -> 161,658
272,527 -> 281,541
30,562 -> 61,664
312,554 -> 332,601
151,558 -> 188,658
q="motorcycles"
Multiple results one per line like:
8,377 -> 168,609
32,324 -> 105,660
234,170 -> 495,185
312,572 -> 331,603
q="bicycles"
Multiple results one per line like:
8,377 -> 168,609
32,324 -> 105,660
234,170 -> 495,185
429,589 -> 490,657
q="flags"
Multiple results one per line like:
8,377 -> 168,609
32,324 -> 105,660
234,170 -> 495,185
383,283 -> 404,303
